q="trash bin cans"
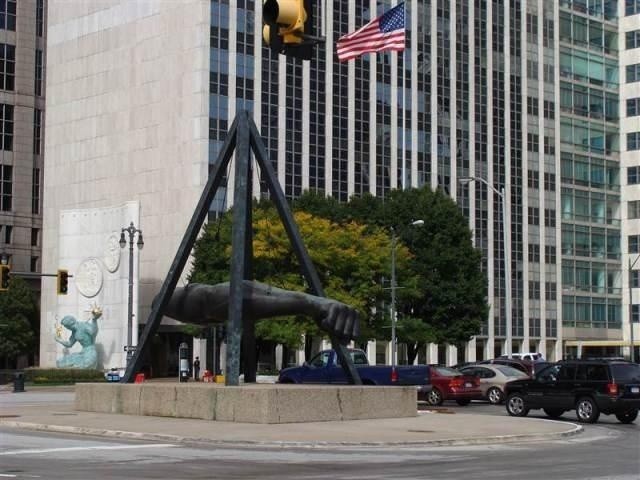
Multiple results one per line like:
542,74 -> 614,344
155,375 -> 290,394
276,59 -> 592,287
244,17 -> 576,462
13,372 -> 25,392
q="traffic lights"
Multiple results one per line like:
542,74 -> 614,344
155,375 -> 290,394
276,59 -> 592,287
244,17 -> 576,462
60,272 -> 68,292
263,0 -> 297,30
2,267 -> 10,288
259,23 -> 271,46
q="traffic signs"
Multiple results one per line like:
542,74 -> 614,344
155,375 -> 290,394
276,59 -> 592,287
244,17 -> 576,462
124,346 -> 140,351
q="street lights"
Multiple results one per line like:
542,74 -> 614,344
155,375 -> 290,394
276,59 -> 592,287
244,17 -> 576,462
120,222 -> 143,375
459,174 -> 511,356
390,220 -> 425,366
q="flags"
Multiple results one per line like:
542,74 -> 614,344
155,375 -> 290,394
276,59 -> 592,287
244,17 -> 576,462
337,3 -> 406,61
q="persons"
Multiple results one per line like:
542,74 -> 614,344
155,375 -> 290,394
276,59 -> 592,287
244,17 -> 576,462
152,279 -> 361,344
54,316 -> 98,369
194,356 -> 201,380
